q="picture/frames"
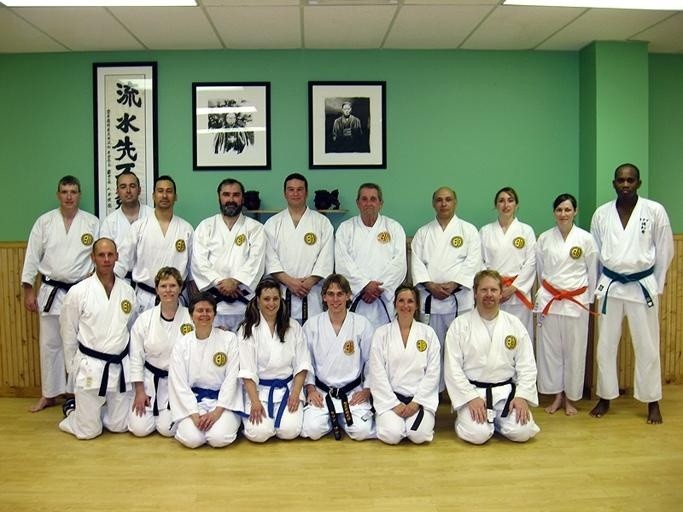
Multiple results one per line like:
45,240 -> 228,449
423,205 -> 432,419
191,80 -> 273,171
307,79 -> 389,175
91,59 -> 158,227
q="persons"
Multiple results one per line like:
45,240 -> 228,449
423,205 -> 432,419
168,292 -> 240,449
126,266 -> 196,437
99,172 -> 155,286
590,163 -> 676,425
368,282 -> 441,446
113,175 -> 194,315
263,173 -> 335,326
238,277 -> 314,443
20,175 -> 99,413
332,102 -> 363,146
536,194 -> 599,417
478,187 -> 537,350
58,238 -> 137,440
300,273 -> 376,441
189,178 -> 267,334
334,183 -> 408,328
444,270 -> 541,445
213,111 -> 248,155
410,186 -> 481,404
